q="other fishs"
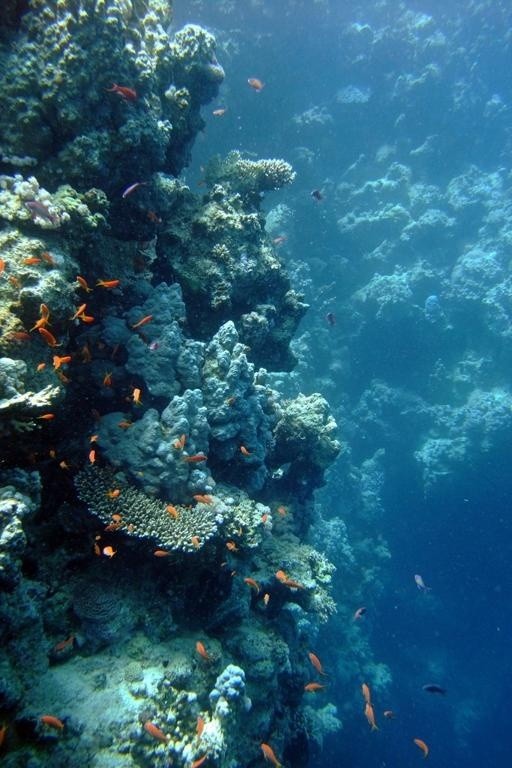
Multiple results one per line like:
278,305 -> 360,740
0,181 -> 303,768
